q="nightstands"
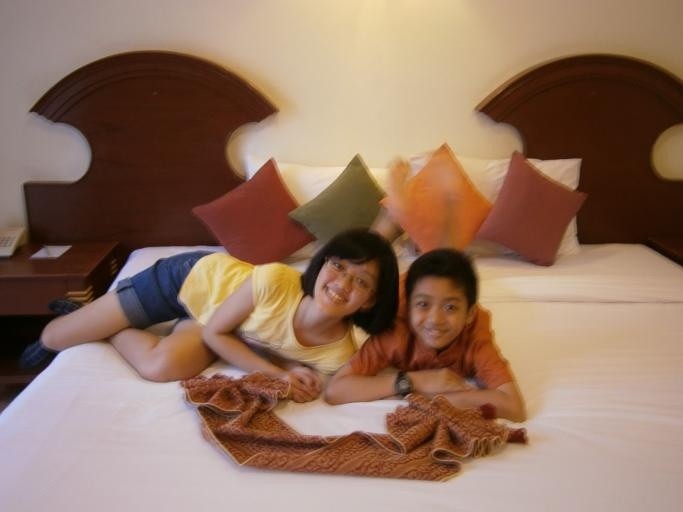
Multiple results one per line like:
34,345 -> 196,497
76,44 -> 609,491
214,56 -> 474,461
648,235 -> 683,265
0,242 -> 121,388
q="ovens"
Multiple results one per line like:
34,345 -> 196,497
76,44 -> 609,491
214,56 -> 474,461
0,50 -> 683,512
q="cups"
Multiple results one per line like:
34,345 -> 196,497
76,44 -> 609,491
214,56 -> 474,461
324,255 -> 377,296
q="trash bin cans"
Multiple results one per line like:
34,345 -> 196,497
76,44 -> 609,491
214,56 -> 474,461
392,370 -> 414,396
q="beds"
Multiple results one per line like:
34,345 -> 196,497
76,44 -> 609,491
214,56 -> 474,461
0,50 -> 683,512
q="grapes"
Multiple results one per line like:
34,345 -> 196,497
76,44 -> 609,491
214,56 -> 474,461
648,235 -> 683,265
0,242 -> 121,388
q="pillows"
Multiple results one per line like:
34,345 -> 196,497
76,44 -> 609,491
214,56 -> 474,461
245,158 -> 394,257
193,157 -> 317,265
380,145 -> 493,254
488,157 -> 583,257
476,151 -> 587,263
287,153 -> 405,251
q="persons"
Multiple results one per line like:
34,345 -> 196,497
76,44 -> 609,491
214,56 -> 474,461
323,157 -> 530,425
9,226 -> 406,405
